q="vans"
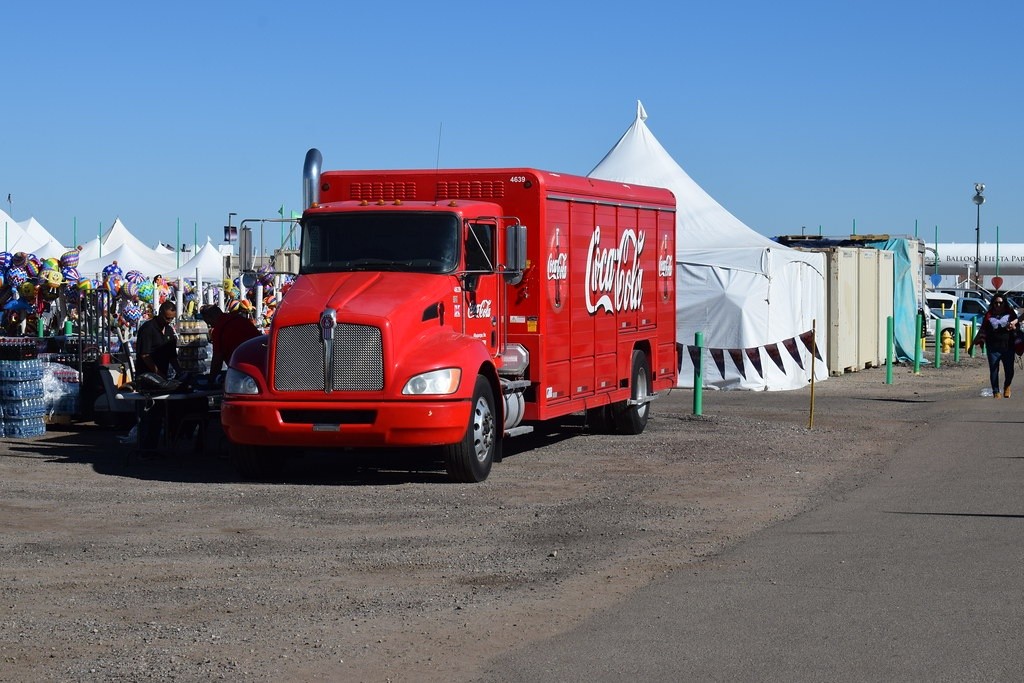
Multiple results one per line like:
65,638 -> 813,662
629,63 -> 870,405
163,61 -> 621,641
938,289 -> 992,306
925,292 -> 960,311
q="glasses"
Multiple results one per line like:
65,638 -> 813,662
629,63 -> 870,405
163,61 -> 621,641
993,301 -> 1004,304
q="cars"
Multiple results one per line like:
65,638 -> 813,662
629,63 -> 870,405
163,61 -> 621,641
927,310 -> 983,349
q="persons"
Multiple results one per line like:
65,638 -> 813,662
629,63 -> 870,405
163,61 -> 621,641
133,301 -> 178,378
201,305 -> 263,388
982,293 -> 1021,399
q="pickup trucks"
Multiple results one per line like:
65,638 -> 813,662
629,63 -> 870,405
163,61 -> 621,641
931,297 -> 990,325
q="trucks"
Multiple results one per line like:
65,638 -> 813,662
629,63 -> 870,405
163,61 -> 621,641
218,145 -> 678,485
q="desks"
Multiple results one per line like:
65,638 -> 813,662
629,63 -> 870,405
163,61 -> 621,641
116,391 -> 211,457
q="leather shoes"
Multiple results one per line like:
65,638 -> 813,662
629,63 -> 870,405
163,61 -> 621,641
993,392 -> 1001,399
1003,385 -> 1011,398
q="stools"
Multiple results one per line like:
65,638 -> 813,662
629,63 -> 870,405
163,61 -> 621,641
172,413 -> 206,455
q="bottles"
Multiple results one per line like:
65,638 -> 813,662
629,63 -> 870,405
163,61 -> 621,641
175,319 -> 210,375
0,336 -> 100,440
